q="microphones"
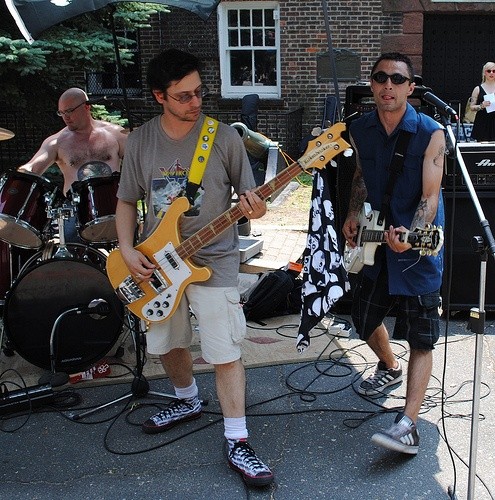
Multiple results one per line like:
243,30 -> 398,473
420,86 -> 458,119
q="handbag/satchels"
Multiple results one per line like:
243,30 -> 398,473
464,97 -> 478,123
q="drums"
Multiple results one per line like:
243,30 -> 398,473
71,176 -> 141,243
2,243 -> 125,374
0,168 -> 66,249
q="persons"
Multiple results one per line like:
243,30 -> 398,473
17,87 -> 132,261
469,62 -> 495,142
116,48 -> 273,486
342,53 -> 446,454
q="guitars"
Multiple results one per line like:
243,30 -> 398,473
340,201 -> 444,274
106,121 -> 349,328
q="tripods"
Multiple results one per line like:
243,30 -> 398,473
71,310 -> 208,419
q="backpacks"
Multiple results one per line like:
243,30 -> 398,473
241,264 -> 306,326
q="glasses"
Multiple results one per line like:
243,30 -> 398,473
486,69 -> 495,73
372,70 -> 413,86
165,84 -> 210,104
56,101 -> 86,117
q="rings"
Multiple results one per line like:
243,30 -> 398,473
136,274 -> 141,279
249,210 -> 252,215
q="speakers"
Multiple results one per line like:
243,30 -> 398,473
443,192 -> 495,313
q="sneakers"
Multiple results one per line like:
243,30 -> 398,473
370,411 -> 420,455
357,358 -> 403,395
142,394 -> 204,435
222,435 -> 274,488
